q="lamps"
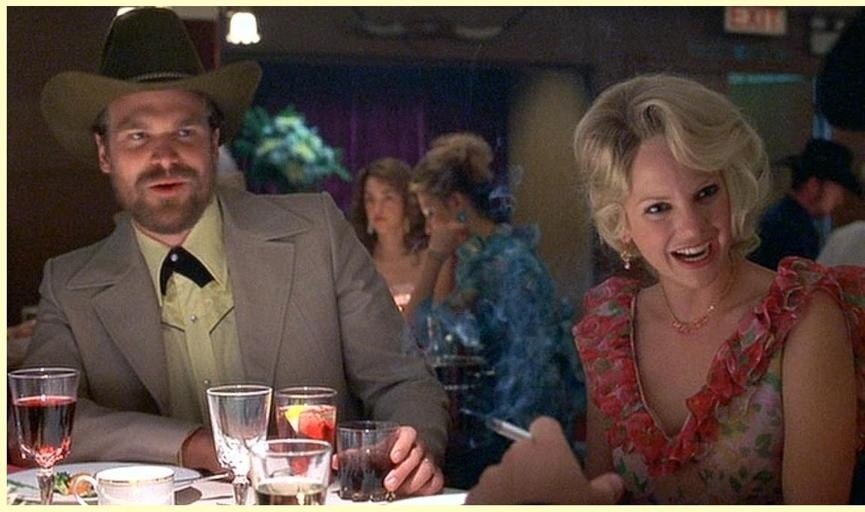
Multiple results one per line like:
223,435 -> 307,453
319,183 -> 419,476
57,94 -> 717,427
223,12 -> 263,45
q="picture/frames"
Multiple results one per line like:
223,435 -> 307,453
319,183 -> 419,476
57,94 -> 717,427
723,7 -> 788,36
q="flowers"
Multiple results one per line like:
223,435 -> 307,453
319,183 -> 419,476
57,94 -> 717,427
233,105 -> 352,191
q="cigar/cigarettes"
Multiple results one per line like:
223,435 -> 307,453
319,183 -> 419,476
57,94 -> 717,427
461,402 -> 537,445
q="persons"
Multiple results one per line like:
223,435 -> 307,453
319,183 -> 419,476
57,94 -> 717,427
461,416 -> 626,504
747,137 -> 865,276
817,12 -> 865,275
571,74 -> 862,505
398,136 -> 587,487
353,158 -> 452,322
6,9 -> 452,502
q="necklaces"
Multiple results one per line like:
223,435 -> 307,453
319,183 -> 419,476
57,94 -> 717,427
654,250 -> 746,334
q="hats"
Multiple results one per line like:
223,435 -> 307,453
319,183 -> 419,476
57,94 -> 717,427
40,7 -> 263,167
779,137 -> 864,197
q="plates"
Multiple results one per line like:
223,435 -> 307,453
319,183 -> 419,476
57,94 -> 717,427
7,462 -> 201,502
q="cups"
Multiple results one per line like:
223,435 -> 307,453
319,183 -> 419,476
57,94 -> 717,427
8,368 -> 80,505
206,385 -> 273,505
251,438 -> 332,505
274,386 -> 338,454
73,465 -> 175,505
337,421 -> 400,501
